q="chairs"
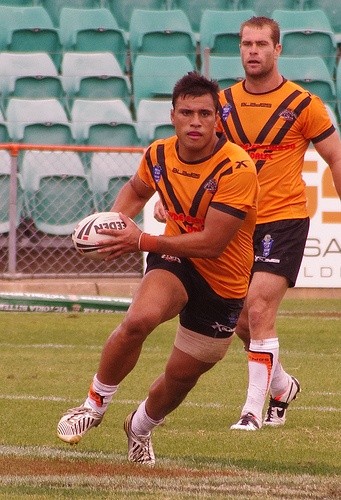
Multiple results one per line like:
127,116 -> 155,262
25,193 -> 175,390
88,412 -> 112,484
0,1 -> 341,235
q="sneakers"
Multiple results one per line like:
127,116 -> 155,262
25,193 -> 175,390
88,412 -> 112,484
123,410 -> 155,468
229,412 -> 262,431
264,375 -> 301,427
57,404 -> 104,445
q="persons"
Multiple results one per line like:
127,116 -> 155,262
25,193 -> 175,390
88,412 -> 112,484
55,72 -> 259,467
212,17 -> 341,430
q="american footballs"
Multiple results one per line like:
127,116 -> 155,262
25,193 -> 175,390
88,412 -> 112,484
71,212 -> 127,261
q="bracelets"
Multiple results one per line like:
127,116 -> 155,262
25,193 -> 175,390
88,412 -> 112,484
137,231 -> 160,252
